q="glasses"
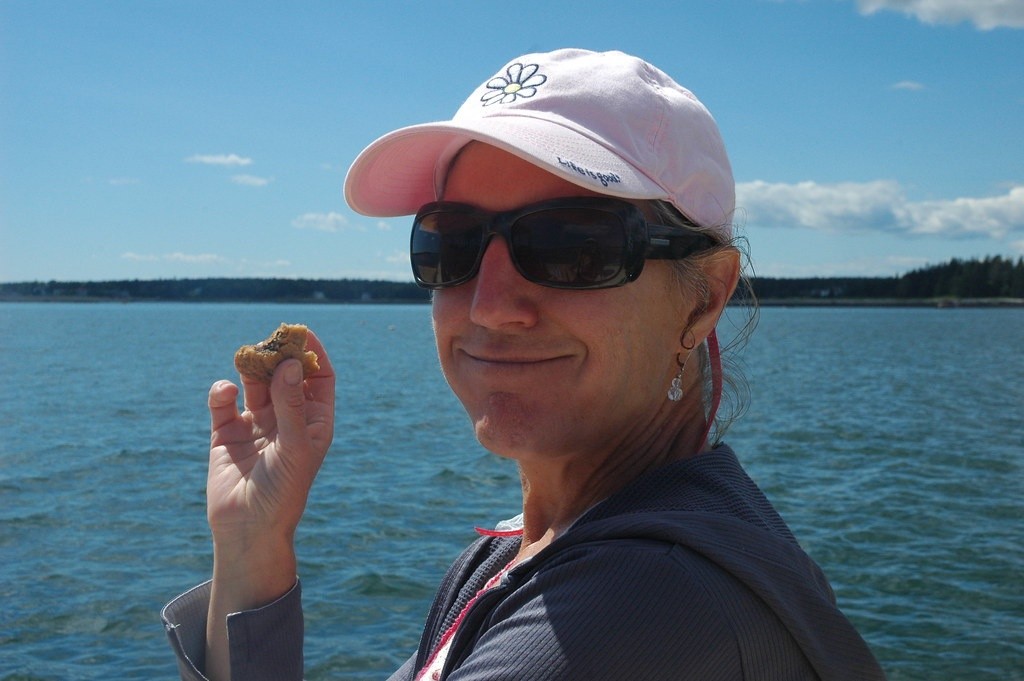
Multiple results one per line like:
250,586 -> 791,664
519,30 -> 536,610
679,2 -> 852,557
410,196 -> 721,290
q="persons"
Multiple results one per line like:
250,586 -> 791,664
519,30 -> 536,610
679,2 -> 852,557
161,48 -> 890,681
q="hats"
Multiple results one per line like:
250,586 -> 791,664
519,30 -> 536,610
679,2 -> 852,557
343,48 -> 736,246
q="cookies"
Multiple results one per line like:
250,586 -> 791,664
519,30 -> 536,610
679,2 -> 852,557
234,322 -> 320,385
